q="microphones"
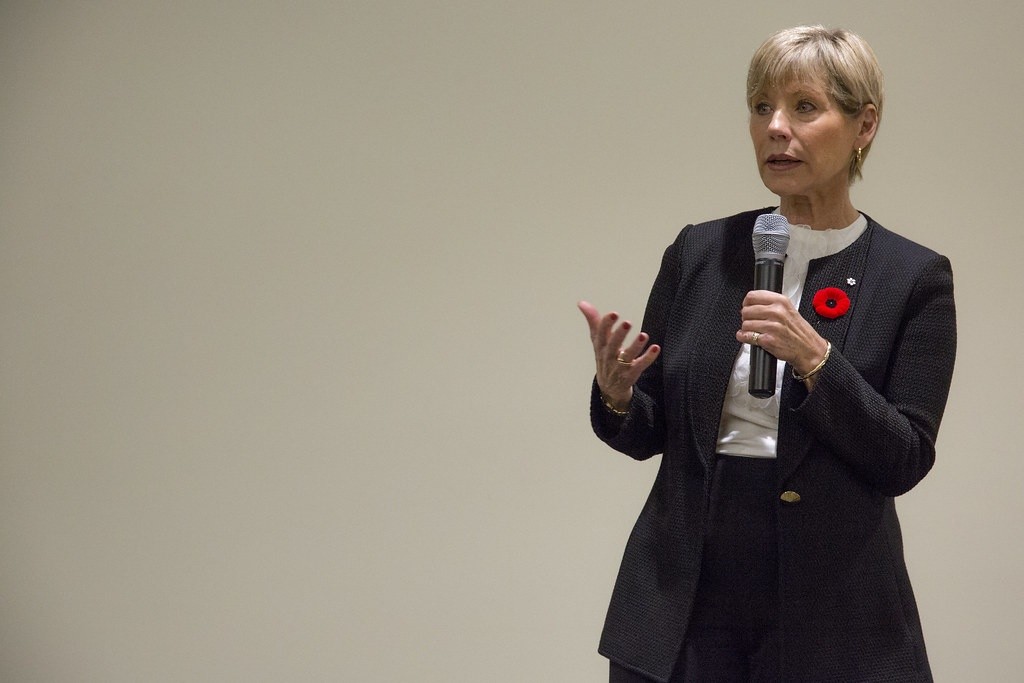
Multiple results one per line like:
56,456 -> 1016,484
748,214 -> 790,399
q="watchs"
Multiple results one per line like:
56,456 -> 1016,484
598,391 -> 629,417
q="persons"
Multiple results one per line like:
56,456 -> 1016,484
573,21 -> 963,683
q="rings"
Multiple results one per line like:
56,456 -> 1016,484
752,332 -> 761,346
615,351 -> 634,367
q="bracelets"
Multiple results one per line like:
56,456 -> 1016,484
791,340 -> 832,381
